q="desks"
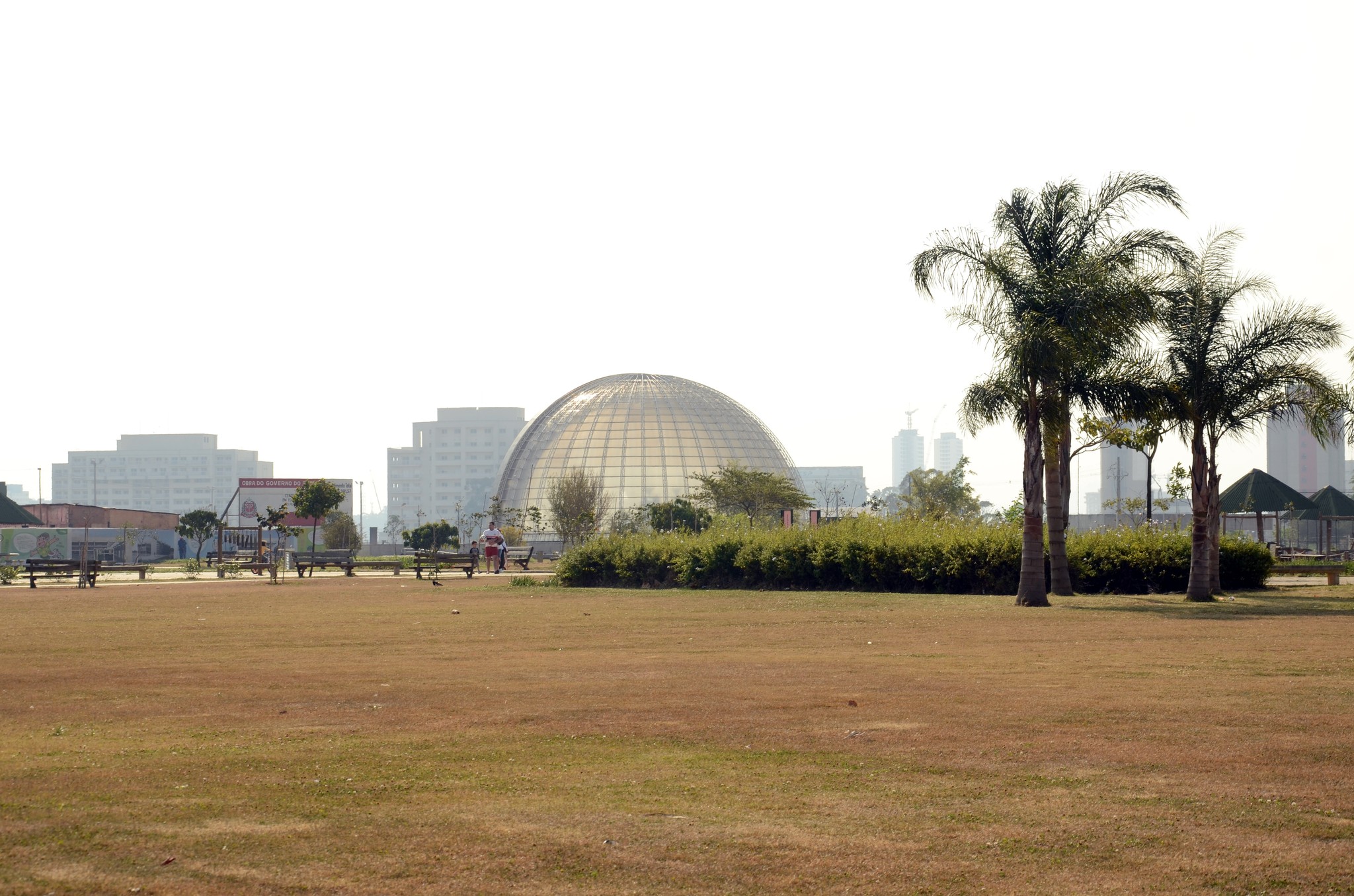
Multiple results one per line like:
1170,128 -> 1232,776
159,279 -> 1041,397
1280,554 -> 1325,561
1282,547 -> 1312,554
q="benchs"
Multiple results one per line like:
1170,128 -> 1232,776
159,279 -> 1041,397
222,555 -> 258,564
485,546 -> 534,570
292,552 -> 359,577
408,552 -> 478,579
98,565 -> 149,579
203,551 -> 237,567
1269,565 -> 1345,585
341,561 -> 403,575
0,553 -> 19,560
23,559 -> 100,589
215,564 -> 275,578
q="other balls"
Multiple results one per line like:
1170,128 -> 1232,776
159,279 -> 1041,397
480,535 -> 487,542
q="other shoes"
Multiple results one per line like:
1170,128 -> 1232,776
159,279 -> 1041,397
504,547 -> 506,553
478,571 -> 482,574
494,570 -> 499,574
498,568 -> 503,573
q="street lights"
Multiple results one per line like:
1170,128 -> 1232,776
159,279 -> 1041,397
37,468 -> 42,521
354,481 -> 364,546
91,459 -> 96,506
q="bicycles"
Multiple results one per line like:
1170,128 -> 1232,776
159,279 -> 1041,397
251,551 -> 275,574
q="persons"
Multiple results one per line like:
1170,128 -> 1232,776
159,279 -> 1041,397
178,536 -> 187,560
479,522 -> 509,574
469,541 -> 482,574
258,540 -> 272,570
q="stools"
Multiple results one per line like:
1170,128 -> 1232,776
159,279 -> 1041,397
19,560 -> 25,566
5,560 -> 12,566
12,560 -> 18,566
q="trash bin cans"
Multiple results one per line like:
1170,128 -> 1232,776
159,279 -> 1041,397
285,551 -> 294,569
